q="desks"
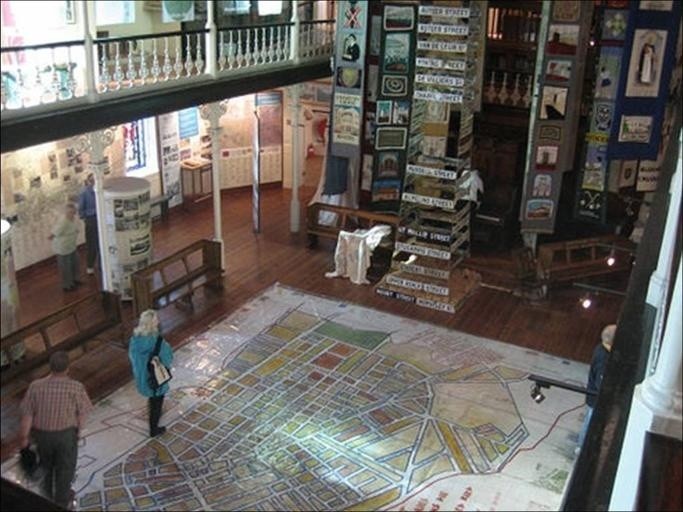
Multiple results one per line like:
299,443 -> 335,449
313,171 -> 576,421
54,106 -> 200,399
179,158 -> 212,209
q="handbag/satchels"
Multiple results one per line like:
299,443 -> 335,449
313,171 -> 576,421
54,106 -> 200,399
146,353 -> 172,390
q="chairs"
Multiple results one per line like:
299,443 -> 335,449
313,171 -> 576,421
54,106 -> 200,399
511,243 -> 549,306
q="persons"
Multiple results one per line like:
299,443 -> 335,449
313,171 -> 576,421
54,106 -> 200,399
47,204 -> 82,291
18,351 -> 93,508
575,324 -> 617,455
128,309 -> 173,436
79,173 -> 101,275
341,34 -> 359,62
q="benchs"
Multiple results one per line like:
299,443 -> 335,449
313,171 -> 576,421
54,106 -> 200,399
538,233 -> 633,291
301,200 -> 401,252
150,194 -> 172,222
129,236 -> 223,320
0,288 -> 127,393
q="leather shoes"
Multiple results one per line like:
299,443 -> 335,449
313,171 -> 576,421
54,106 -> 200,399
150,427 -> 165,437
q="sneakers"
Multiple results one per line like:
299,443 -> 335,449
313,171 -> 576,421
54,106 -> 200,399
63,267 -> 94,292
